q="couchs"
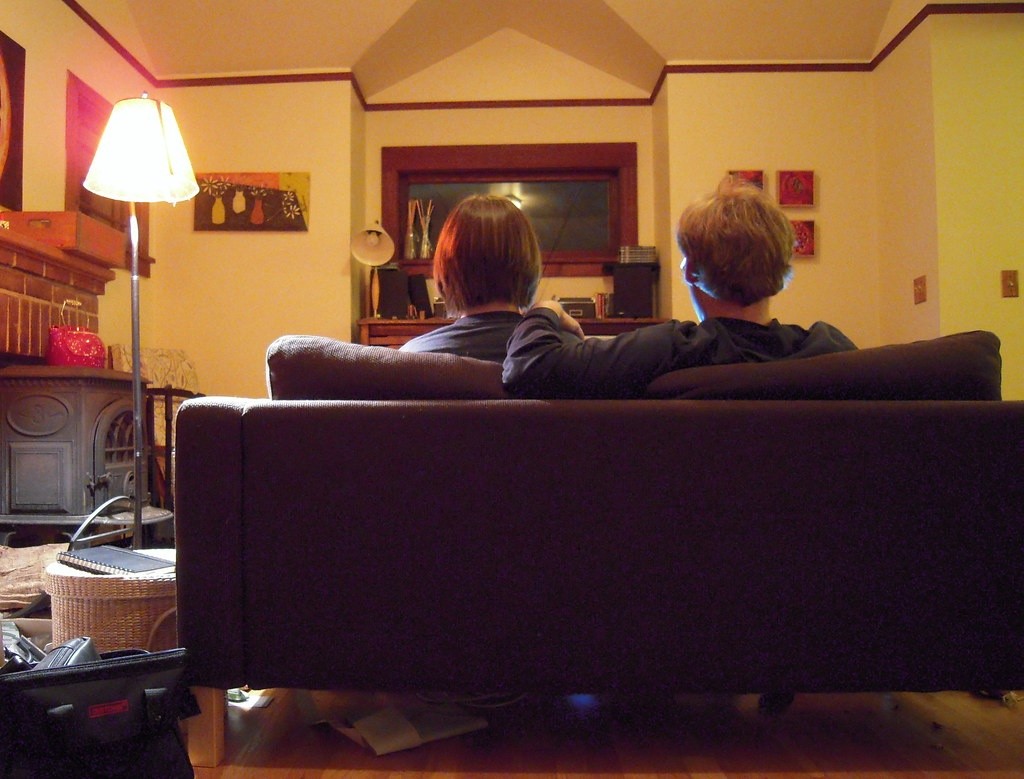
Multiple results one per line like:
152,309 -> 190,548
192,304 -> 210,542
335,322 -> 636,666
176,398 -> 1024,769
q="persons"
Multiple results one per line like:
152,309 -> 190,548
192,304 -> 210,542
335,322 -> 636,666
397,195 -> 580,362
501,183 -> 859,394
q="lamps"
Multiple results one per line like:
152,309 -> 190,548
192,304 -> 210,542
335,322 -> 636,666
351,222 -> 394,318
84,91 -> 200,547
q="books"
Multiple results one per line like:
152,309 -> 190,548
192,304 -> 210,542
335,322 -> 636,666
56,546 -> 176,578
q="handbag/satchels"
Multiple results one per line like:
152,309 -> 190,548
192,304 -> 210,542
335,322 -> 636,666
0,647 -> 201,779
47,300 -> 106,368
33,636 -> 103,669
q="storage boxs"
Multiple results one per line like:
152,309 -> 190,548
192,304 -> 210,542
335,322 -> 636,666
554,245 -> 657,319
1,210 -> 126,268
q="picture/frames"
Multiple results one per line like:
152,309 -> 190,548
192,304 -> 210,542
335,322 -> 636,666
775,170 -> 816,206
791,219 -> 816,259
726,169 -> 765,190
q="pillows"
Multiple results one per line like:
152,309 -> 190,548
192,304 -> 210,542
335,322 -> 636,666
266,335 -> 505,401
647,329 -> 1001,401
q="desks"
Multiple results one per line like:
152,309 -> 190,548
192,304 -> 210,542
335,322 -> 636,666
357,319 -> 669,350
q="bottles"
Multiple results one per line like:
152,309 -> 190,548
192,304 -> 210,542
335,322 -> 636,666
420,215 -> 435,259
404,223 -> 420,259
